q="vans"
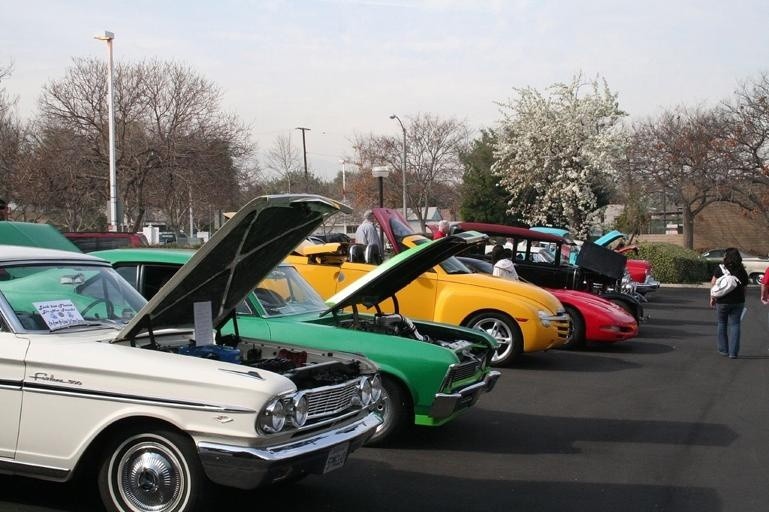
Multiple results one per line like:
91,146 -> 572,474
63,233 -> 150,255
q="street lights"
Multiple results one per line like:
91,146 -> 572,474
390,113 -> 408,223
94,29 -> 122,234
295,126 -> 312,191
339,160 -> 349,235
371,165 -> 389,260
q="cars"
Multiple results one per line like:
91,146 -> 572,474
425,223 -> 661,333
1,222 -> 502,445
1,192 -> 387,511
701,248 -> 769,285
222,211 -> 575,366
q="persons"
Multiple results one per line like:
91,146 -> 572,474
0,199 -> 10,223
760,265 -> 769,305
709,246 -> 744,359
354,207 -> 381,257
614,239 -> 645,257
432,218 -> 451,239
488,244 -> 517,284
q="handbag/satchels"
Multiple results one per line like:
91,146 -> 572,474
711,264 -> 741,298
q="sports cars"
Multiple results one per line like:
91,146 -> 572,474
372,206 -> 640,349
